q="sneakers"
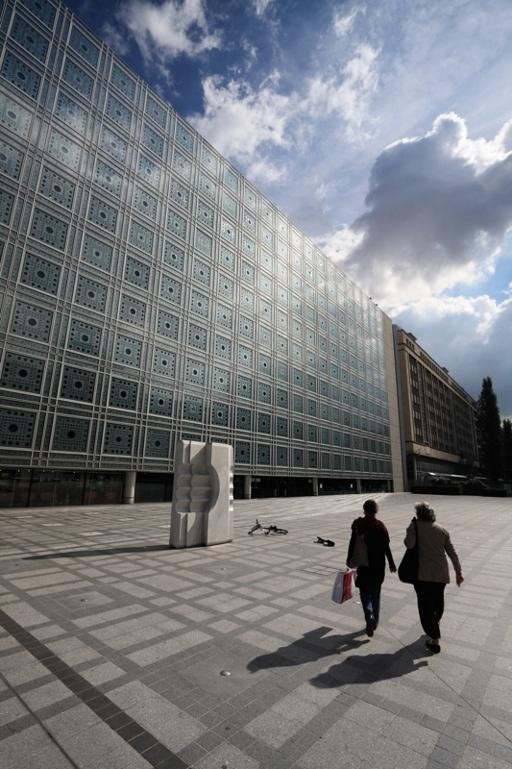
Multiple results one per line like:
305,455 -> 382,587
366,614 -> 375,637
425,639 -> 440,654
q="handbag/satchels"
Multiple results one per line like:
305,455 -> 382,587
349,536 -> 368,567
330,570 -> 355,604
397,548 -> 416,583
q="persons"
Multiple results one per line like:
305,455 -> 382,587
404,502 -> 464,654
346,499 -> 396,637
